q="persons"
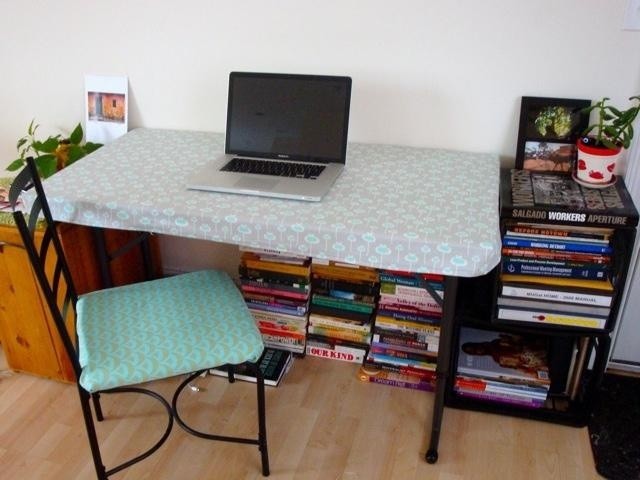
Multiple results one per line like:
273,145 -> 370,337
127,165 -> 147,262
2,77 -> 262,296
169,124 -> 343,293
461,333 -> 549,371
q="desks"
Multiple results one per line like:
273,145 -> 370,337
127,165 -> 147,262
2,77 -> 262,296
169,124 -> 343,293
24,127 -> 502,465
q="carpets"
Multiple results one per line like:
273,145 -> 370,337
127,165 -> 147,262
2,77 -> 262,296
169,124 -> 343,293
586,371 -> 639,479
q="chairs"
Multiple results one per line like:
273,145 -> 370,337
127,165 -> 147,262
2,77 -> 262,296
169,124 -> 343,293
7,155 -> 269,479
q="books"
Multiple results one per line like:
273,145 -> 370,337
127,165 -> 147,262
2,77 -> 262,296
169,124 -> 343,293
501,220 -> 613,291
497,287 -> 612,329
500,170 -> 639,228
193,244 -> 445,393
455,327 -> 550,408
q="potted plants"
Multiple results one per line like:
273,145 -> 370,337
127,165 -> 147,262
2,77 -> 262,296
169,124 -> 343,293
569,95 -> 640,189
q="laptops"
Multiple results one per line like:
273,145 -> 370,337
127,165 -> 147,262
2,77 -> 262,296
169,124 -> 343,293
185,72 -> 352,202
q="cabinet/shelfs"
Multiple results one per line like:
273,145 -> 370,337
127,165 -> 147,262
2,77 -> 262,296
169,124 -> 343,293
0,221 -> 154,386
444,218 -> 640,430
